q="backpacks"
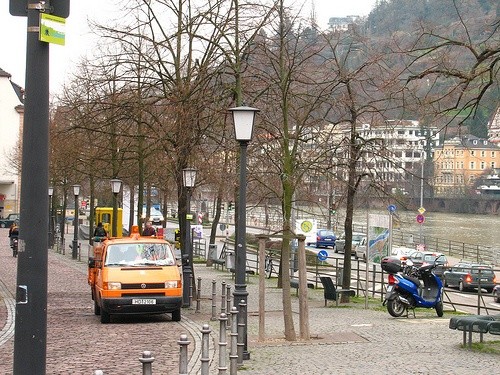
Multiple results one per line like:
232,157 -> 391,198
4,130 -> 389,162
97,228 -> 105,236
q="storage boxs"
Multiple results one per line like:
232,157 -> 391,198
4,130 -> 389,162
381,256 -> 401,273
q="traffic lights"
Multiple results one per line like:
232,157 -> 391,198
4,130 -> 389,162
332,204 -> 335,213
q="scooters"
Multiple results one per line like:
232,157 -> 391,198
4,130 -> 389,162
380,253 -> 445,318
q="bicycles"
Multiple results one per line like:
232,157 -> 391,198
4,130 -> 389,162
8,234 -> 18,258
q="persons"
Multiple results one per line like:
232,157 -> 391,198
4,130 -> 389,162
8,223 -> 19,255
142,223 -> 156,237
94,222 -> 107,242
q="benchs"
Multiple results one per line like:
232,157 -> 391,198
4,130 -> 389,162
229,267 -> 255,282
289,278 -> 314,297
316,275 -> 355,306
210,258 -> 226,271
450,313 -> 500,347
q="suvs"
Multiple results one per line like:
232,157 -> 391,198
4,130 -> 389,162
441,262 -> 496,292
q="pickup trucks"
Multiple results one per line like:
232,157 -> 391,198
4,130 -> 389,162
88,241 -> 189,324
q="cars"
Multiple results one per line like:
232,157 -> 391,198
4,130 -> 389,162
408,250 -> 450,279
333,231 -> 367,255
0,213 -> 20,228
143,206 -> 164,224
307,229 -> 336,248
492,285 -> 500,303
355,236 -> 367,263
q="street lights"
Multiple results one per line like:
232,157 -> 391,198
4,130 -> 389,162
48,187 -> 55,245
71,184 -> 82,258
110,178 -> 123,237
181,168 -> 198,306
228,106 -> 260,360
